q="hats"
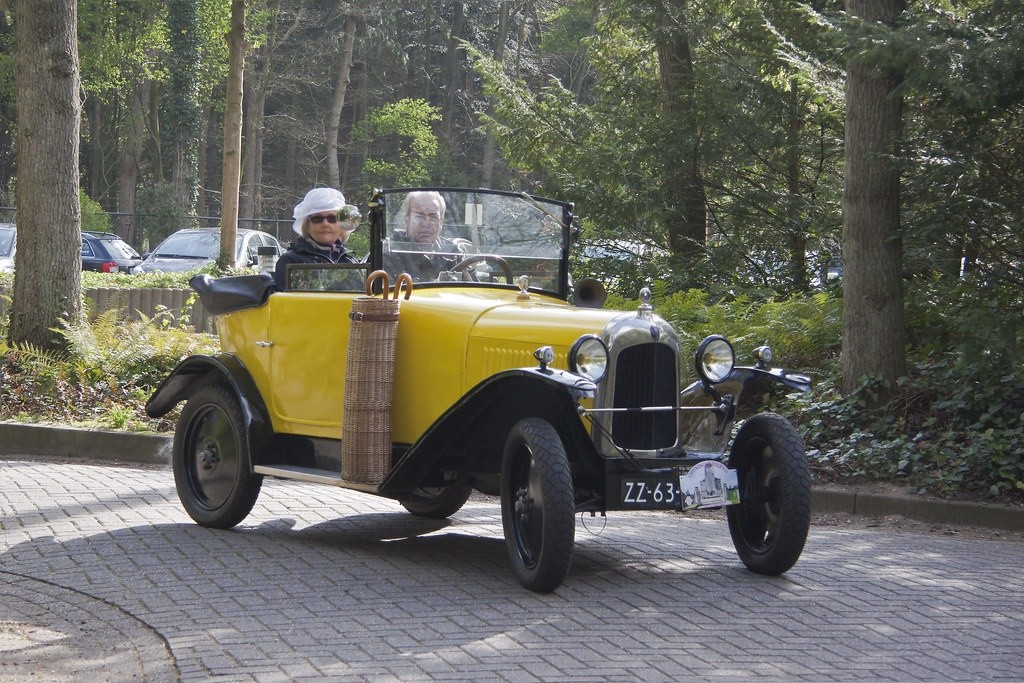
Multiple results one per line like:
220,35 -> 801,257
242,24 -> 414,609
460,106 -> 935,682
293,188 -> 345,218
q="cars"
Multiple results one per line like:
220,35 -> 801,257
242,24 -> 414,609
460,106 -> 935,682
132,226 -> 287,276
0,221 -> 18,277
145,186 -> 814,596
82,231 -> 143,274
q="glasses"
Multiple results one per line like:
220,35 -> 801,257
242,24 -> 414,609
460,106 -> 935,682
309,213 -> 337,224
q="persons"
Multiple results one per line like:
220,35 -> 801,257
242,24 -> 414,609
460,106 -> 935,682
274,187 -> 368,291
361,190 -> 481,290
